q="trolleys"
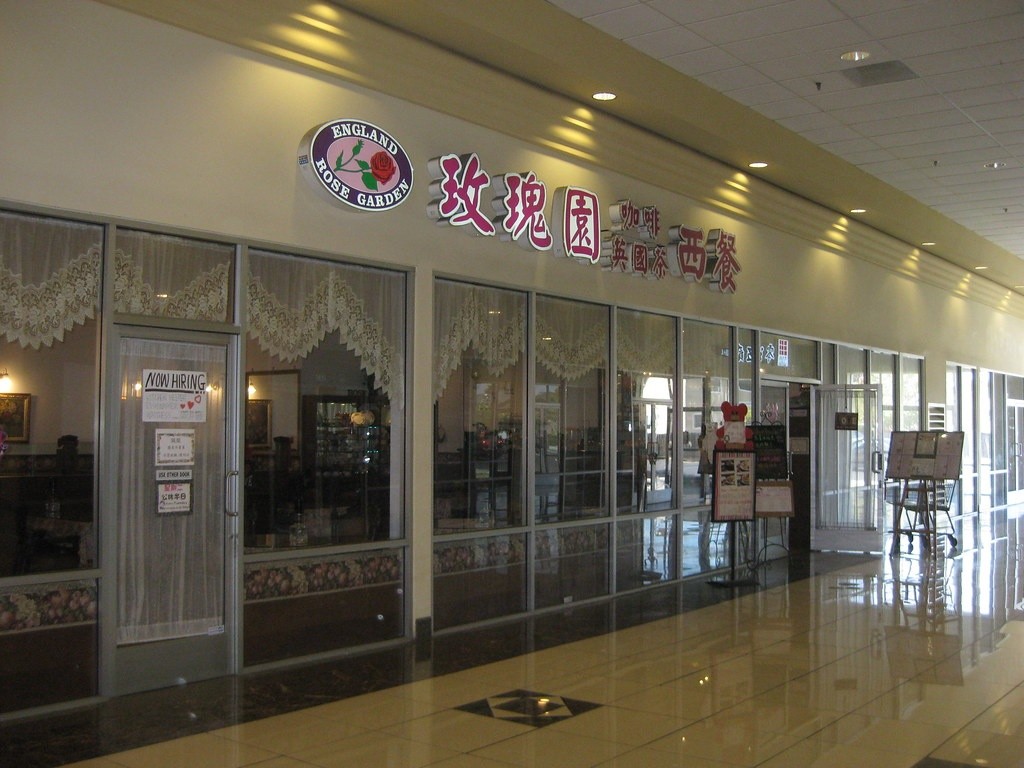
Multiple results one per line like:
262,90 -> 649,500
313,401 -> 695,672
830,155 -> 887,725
883,477 -> 958,547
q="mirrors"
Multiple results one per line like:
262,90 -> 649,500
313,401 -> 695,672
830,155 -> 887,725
246,369 -> 302,457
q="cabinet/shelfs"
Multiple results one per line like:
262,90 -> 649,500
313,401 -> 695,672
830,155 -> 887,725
302,394 -> 365,489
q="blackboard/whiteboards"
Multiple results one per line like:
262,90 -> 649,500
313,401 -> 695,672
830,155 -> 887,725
756,481 -> 795,518
711,449 -> 758,522
745,426 -> 788,479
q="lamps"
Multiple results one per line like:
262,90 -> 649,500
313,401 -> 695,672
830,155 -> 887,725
248,375 -> 256,394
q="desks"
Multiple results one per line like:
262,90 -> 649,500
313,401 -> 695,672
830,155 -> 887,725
0,470 -> 93,518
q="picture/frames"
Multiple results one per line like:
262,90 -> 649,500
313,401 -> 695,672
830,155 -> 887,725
711,449 -> 757,523
248,399 -> 272,449
0,392 -> 30,443
